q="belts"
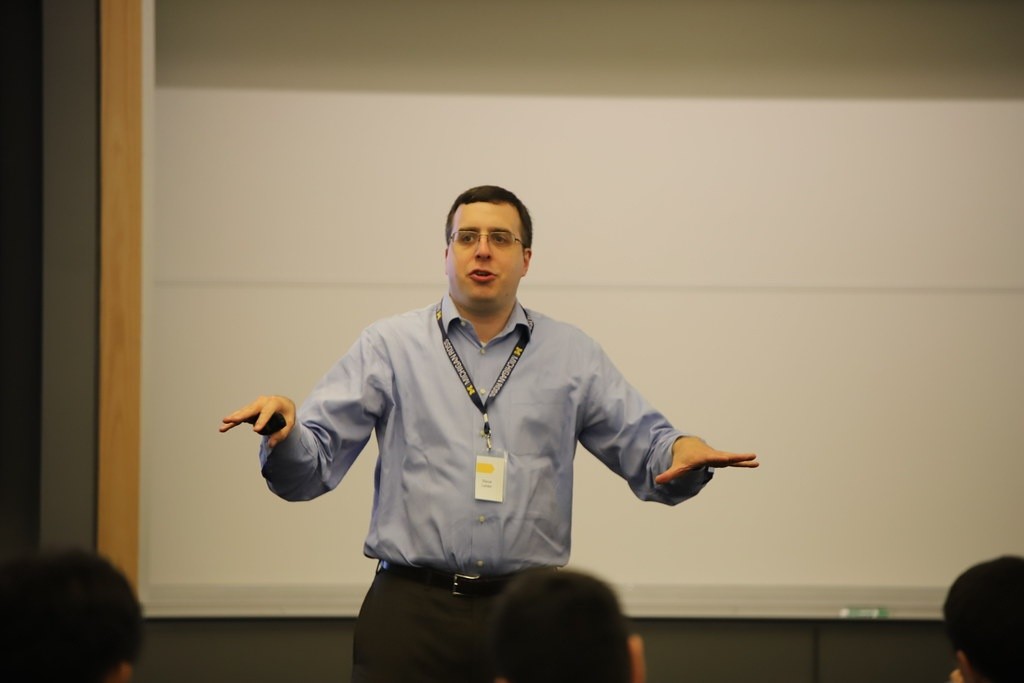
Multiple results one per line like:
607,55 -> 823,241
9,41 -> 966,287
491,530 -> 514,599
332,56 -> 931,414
378,561 -> 558,599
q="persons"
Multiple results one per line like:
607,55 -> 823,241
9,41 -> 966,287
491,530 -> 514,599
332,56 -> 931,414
942,554 -> 1024,683
220,185 -> 760,683
0,546 -> 143,683
494,570 -> 646,682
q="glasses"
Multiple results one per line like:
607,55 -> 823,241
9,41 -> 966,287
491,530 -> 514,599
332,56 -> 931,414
447,231 -> 526,249
